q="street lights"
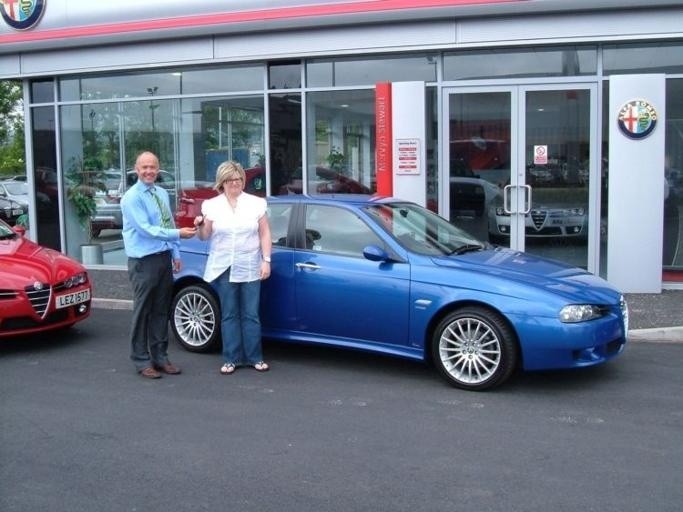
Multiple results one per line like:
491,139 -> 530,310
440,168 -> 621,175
145,86 -> 161,168
88,109 -> 99,169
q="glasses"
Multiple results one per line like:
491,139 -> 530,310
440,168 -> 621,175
223,178 -> 241,184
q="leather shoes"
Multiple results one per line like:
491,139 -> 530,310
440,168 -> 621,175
142,367 -> 161,379
163,363 -> 181,373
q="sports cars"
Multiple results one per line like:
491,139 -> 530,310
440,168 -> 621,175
170,193 -> 629,393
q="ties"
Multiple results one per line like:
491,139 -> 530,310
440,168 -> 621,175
148,188 -> 172,229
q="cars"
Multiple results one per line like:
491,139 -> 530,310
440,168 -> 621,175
0,174 -> 27,182
0,181 -> 27,215
36,191 -> 53,216
0,197 -> 24,228
432,176 -> 587,246
36,166 -> 113,238
0,219 -> 91,338
483,160 -> 605,246
449,158 -> 485,218
173,165 -> 438,239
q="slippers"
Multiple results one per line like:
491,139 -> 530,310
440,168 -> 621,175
254,361 -> 269,372
221,363 -> 235,375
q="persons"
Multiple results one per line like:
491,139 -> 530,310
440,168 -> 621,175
193,161 -> 271,374
120,151 -> 197,379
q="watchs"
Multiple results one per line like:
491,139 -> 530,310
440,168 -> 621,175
262,256 -> 271,263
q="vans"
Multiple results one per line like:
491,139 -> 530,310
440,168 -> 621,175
84,169 -> 174,239
